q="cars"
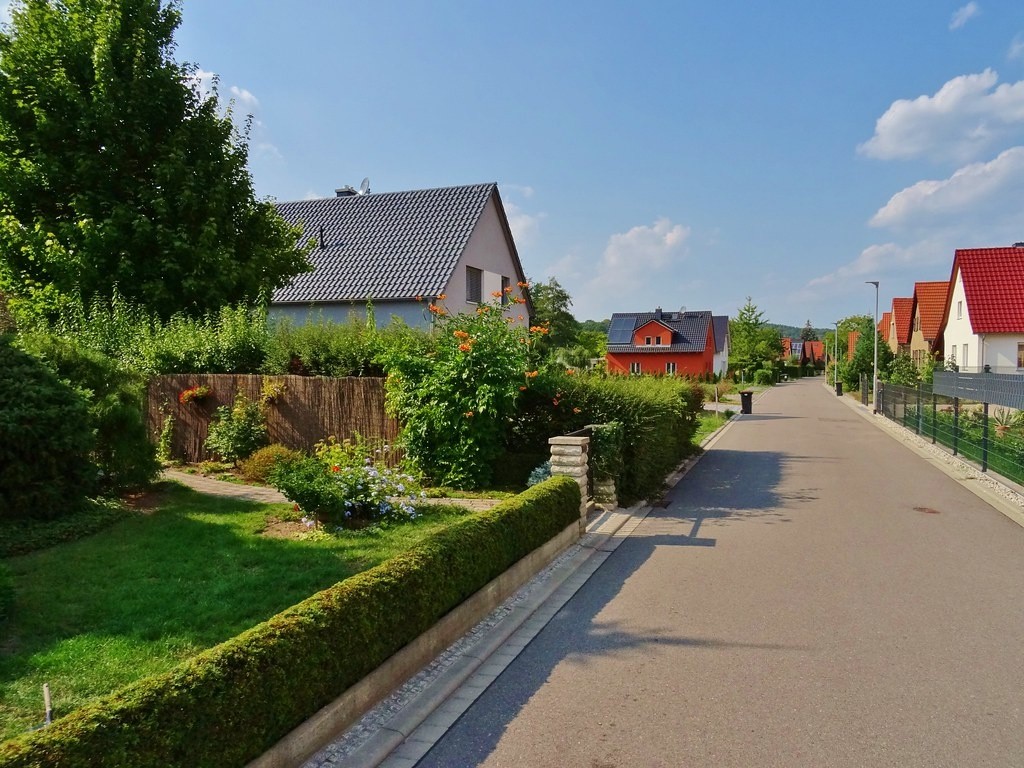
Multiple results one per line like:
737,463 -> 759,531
780,371 -> 788,381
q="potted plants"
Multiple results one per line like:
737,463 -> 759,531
993,406 -> 1013,438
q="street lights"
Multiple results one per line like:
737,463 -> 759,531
831,322 -> 838,392
866,281 -> 879,415
825,337 -> 831,384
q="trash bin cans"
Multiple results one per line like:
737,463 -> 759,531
783,374 -> 788,381
739,390 -> 754,414
835,381 -> 844,396
